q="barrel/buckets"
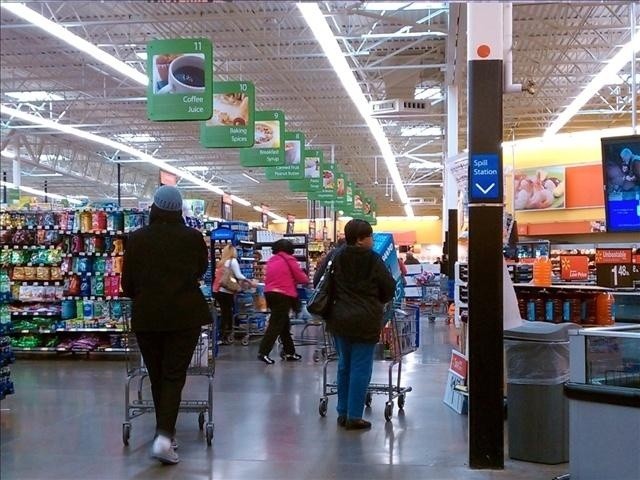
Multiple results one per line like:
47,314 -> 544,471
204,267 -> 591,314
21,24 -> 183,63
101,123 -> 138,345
514,288 -> 616,326
532,246 -> 553,285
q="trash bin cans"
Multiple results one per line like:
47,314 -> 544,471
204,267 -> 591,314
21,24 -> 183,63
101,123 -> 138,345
504,321 -> 583,465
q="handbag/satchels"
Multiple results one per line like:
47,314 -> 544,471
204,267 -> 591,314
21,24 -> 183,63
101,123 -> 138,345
307,248 -> 341,316
292,298 -> 301,312
219,258 -> 239,292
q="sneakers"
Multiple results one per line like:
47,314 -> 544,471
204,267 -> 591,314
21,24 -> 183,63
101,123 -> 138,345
283,353 -> 301,360
337,416 -> 371,430
257,354 -> 274,364
217,340 -> 231,345
150,436 -> 180,463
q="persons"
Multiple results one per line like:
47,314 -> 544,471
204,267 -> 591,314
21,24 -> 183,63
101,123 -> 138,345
404,252 -> 420,264
212,244 -> 258,345
323,218 -> 398,431
257,238 -> 310,364
433,257 -> 441,264
313,237 -> 346,288
316,251 -> 328,271
606,147 -> 640,190
121,184 -> 216,465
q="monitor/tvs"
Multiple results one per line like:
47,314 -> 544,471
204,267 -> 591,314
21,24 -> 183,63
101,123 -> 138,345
599,134 -> 640,234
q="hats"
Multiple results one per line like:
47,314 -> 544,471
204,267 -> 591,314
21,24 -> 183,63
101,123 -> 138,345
154,186 -> 183,212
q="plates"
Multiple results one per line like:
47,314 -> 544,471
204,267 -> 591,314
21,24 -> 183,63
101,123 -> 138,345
536,173 -> 565,208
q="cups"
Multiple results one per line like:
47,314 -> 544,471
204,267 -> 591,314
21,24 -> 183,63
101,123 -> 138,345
157,55 -> 205,95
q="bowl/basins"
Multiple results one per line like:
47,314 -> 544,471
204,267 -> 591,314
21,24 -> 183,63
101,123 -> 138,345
322,171 -> 334,187
284,143 -> 296,164
253,122 -> 274,148
304,160 -> 316,177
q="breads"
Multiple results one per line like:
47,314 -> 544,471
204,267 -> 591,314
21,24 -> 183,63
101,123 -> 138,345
156,55 -> 178,82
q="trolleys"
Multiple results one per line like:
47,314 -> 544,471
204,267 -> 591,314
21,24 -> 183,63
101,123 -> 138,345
319,297 -> 419,422
120,300 -> 216,447
225,283 -> 323,362
